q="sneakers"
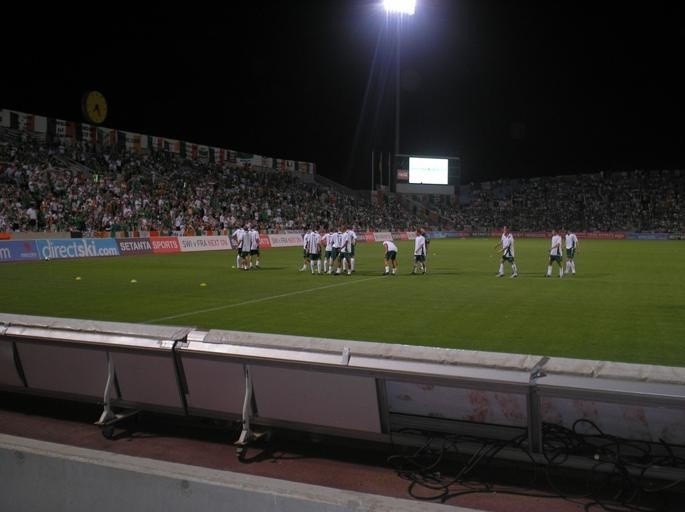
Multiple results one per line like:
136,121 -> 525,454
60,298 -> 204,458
299,268 -> 354,275
497,274 -> 519,278
409,272 -> 426,275
383,272 -> 396,276
237,264 -> 260,271
545,273 -> 576,278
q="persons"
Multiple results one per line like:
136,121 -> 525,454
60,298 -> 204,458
0,125 -> 685,240
410,227 -> 427,275
417,228 -> 430,270
563,229 -> 578,274
543,228 -> 564,278
230,222 -> 359,277
382,239 -> 398,275
493,225 -> 519,278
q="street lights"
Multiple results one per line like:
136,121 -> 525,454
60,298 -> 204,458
382,0 -> 420,184
